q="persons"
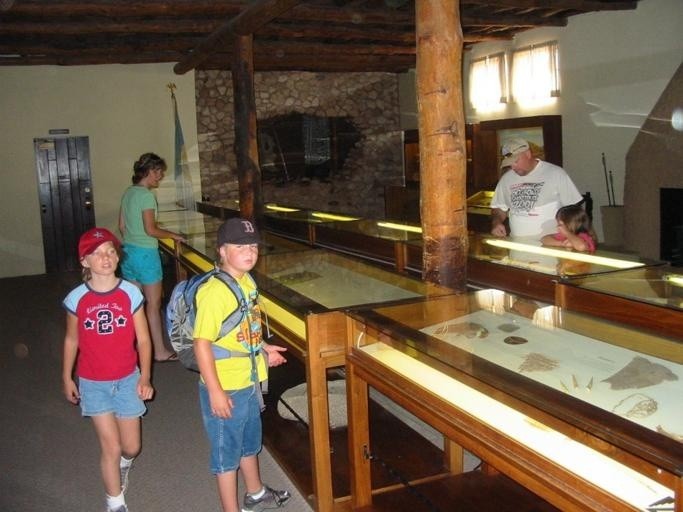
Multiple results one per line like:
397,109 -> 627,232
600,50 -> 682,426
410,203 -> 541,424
490,136 -> 585,244
62,228 -> 155,512
118,152 -> 188,362
502,292 -> 555,319
557,258 -> 590,287
540,204 -> 597,253
192,217 -> 292,512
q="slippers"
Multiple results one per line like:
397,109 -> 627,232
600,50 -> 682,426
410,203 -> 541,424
153,351 -> 179,363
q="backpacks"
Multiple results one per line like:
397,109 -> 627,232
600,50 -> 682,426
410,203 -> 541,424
165,267 -> 247,373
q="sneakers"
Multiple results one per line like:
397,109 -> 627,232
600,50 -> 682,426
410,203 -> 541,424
106,462 -> 131,512
243,484 -> 292,511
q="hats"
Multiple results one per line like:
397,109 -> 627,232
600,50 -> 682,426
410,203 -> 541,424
217,218 -> 260,245
79,228 -> 121,258
500,139 -> 529,168
